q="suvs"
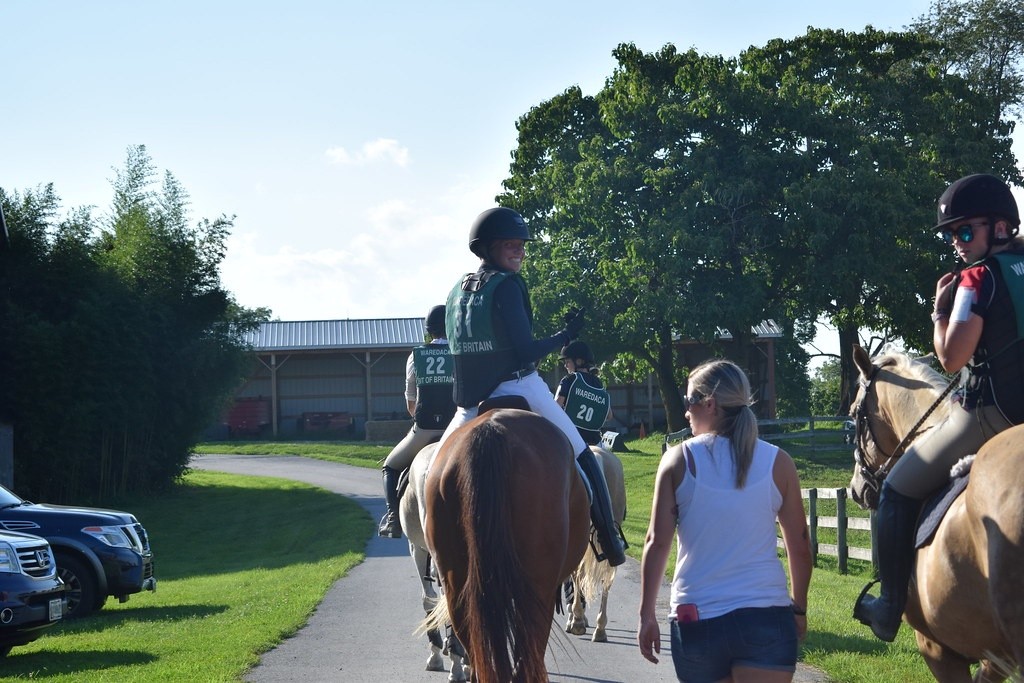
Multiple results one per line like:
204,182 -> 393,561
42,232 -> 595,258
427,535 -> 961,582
1,486 -> 157,618
0,528 -> 68,659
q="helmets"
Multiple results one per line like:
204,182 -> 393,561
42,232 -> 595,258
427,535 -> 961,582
929,174 -> 1021,233
558,341 -> 592,362
425,304 -> 448,335
468,208 -> 537,257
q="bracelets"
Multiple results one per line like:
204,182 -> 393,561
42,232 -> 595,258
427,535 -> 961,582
795,611 -> 806,615
930,309 -> 949,323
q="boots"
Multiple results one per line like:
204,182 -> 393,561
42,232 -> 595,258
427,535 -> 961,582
575,443 -> 629,566
859,481 -> 924,643
377,464 -> 401,538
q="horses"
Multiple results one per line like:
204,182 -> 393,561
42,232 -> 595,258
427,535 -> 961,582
849,341 -> 1024,683
398,407 -> 626,683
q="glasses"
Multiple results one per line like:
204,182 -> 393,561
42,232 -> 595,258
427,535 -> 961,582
684,395 -> 710,410
942,218 -> 1002,244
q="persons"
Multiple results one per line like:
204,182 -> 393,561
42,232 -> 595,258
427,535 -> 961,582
856,174 -> 1024,641
425,208 -> 626,567
554,339 -> 612,448
637,361 -> 813,683
378,306 -> 457,540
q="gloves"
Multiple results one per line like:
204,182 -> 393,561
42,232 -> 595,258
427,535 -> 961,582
561,306 -> 585,342
